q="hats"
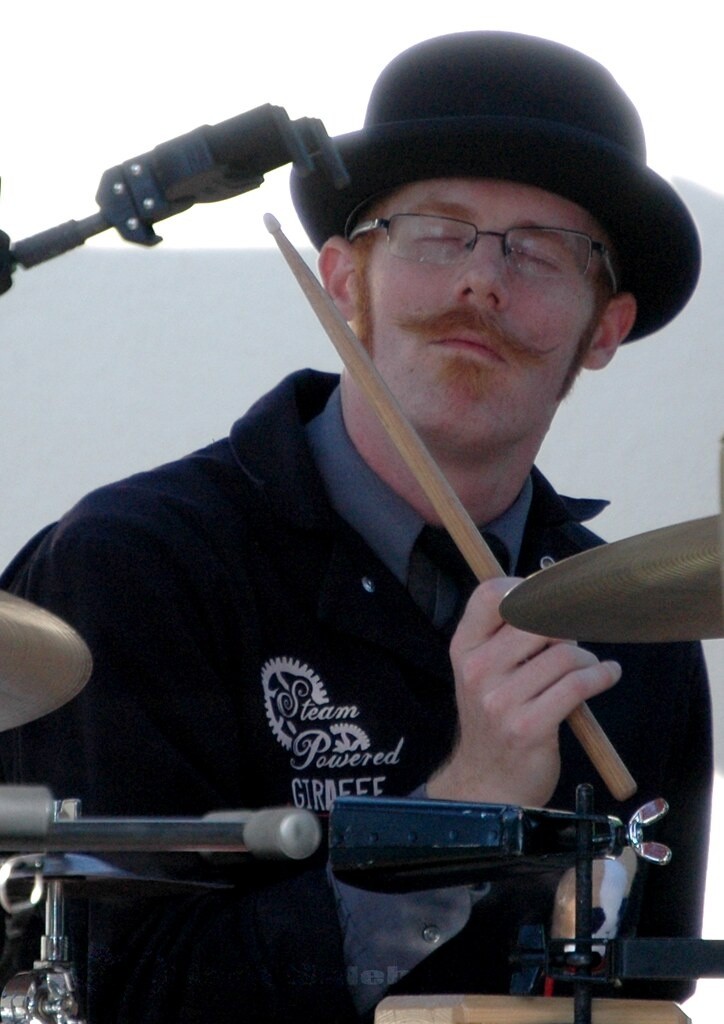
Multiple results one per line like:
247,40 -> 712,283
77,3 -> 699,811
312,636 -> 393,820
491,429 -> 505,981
290,30 -> 702,345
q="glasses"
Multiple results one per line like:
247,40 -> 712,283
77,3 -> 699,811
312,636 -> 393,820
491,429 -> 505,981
350,212 -> 618,299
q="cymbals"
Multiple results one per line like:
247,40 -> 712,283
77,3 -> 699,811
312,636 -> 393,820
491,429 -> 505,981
1,590 -> 95,731
497,509 -> 724,643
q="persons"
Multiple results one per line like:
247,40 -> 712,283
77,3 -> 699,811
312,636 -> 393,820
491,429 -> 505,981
0,17 -> 713,1024
